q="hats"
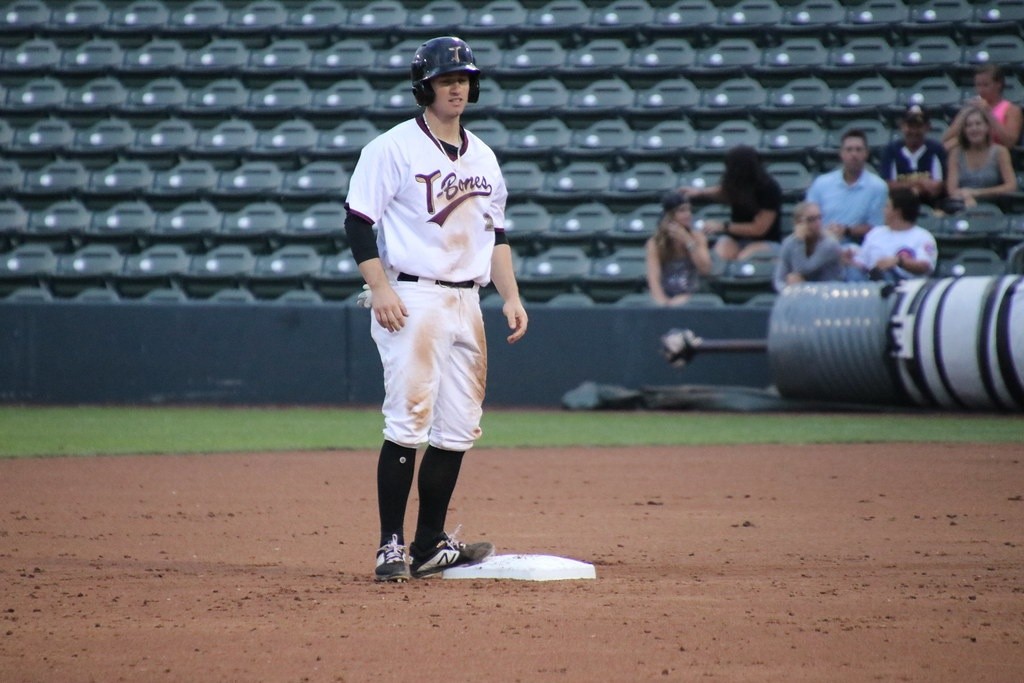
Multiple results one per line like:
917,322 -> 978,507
901,102 -> 930,128
657,192 -> 689,225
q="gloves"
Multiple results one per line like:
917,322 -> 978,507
357,283 -> 372,309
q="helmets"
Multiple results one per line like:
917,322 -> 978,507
411,36 -> 480,106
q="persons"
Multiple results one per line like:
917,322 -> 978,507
879,106 -> 949,207
941,101 -> 1017,208
943,62 -> 1022,150
841,185 -> 937,284
803,129 -> 889,282
683,145 -> 783,259
645,190 -> 711,305
770,203 -> 842,296
345,37 -> 529,583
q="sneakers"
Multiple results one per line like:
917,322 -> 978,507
375,533 -> 409,583
408,524 -> 495,579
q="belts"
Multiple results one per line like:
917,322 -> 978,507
397,272 -> 474,289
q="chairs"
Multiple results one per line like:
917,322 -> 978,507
0,0 -> 1023,306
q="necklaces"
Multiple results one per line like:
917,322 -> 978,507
420,111 -> 461,172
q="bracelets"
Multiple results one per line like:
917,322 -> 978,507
897,255 -> 903,267
722,220 -> 730,234
844,225 -> 851,238
